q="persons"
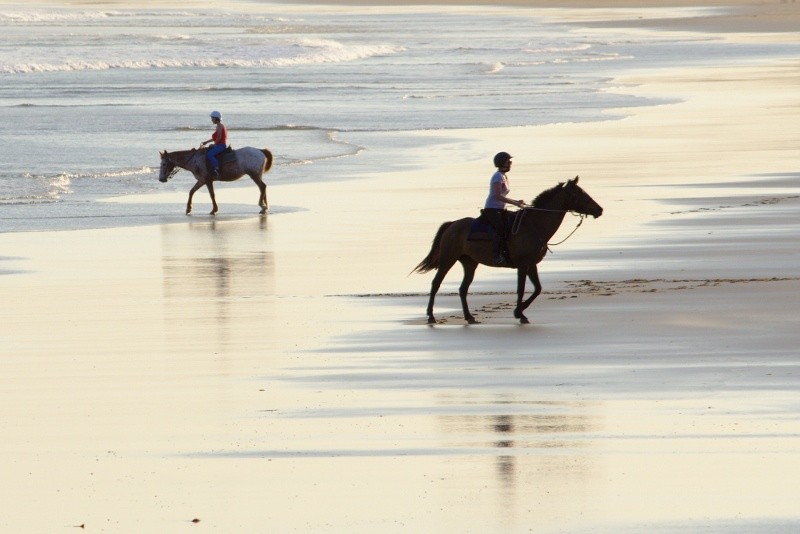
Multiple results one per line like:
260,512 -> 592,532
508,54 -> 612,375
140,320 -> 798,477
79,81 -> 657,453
200,110 -> 227,176
482,152 -> 526,263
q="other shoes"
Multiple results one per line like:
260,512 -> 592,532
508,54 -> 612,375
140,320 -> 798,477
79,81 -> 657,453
210,169 -> 220,175
496,257 -> 511,266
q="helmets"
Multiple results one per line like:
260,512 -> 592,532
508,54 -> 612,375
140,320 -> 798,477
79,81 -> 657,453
210,111 -> 222,120
494,152 -> 512,167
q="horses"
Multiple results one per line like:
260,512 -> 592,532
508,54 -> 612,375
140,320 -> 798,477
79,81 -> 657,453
407,176 -> 603,324
158,147 -> 273,215
